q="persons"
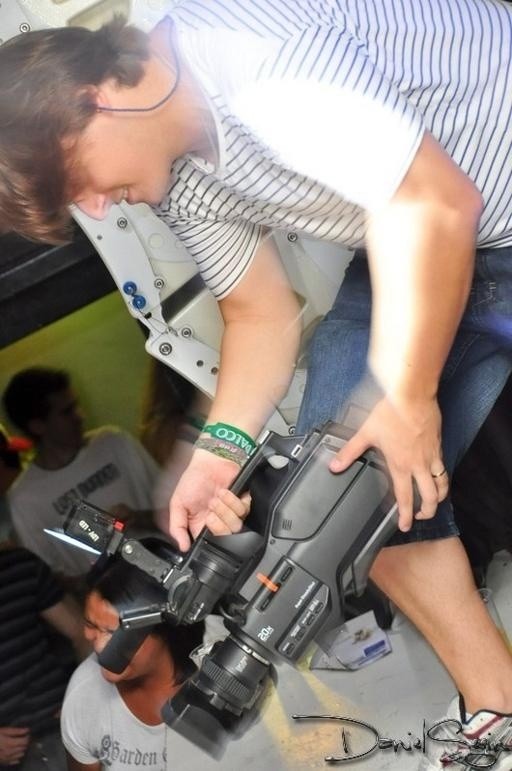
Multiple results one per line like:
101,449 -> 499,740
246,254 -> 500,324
1,366 -> 161,610
2,0 -> 512,770
54,537 -> 235,771
3,541 -> 97,771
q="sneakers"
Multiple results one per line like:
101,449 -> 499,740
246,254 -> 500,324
413,692 -> 512,771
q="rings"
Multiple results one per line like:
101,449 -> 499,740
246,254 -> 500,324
432,469 -> 446,478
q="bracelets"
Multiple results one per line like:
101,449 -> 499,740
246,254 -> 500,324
192,438 -> 248,468
202,422 -> 257,457
